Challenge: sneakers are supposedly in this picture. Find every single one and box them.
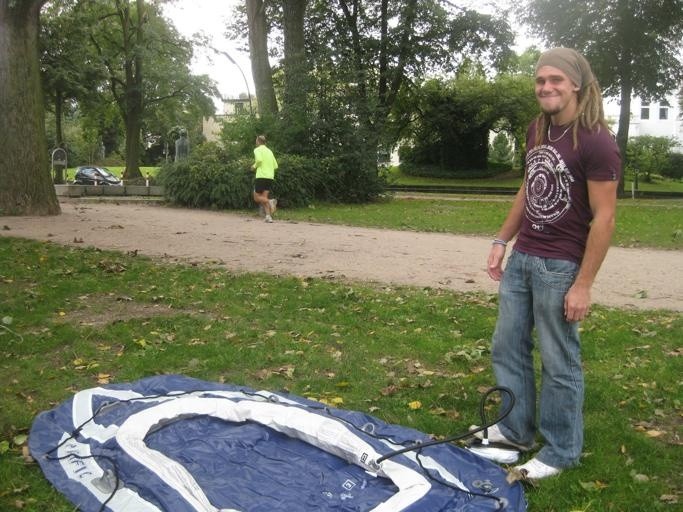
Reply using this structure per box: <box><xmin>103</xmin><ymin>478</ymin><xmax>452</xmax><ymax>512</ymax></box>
<box><xmin>514</xmin><ymin>458</ymin><xmax>561</xmax><ymax>481</ymax></box>
<box><xmin>469</xmin><ymin>423</ymin><xmax>530</xmax><ymax>452</ymax></box>
<box><xmin>265</xmin><ymin>216</ymin><xmax>273</xmax><ymax>223</ymax></box>
<box><xmin>269</xmin><ymin>199</ymin><xmax>277</xmax><ymax>214</ymax></box>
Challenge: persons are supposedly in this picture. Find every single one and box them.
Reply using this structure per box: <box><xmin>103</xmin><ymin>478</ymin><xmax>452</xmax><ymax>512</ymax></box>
<box><xmin>251</xmin><ymin>136</ymin><xmax>278</xmax><ymax>223</ymax></box>
<box><xmin>468</xmin><ymin>47</ymin><xmax>622</xmax><ymax>479</ymax></box>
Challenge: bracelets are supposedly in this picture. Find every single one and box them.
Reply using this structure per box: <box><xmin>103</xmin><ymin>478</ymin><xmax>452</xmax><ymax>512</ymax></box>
<box><xmin>492</xmin><ymin>238</ymin><xmax>508</xmax><ymax>246</ymax></box>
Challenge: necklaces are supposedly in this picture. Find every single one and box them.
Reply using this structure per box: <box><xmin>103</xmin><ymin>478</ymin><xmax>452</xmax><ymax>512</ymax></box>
<box><xmin>547</xmin><ymin>119</ymin><xmax>576</xmax><ymax>143</ymax></box>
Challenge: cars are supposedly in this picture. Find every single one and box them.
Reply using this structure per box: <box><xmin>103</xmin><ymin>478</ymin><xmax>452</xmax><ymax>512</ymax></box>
<box><xmin>73</xmin><ymin>166</ymin><xmax>123</xmax><ymax>187</ymax></box>
